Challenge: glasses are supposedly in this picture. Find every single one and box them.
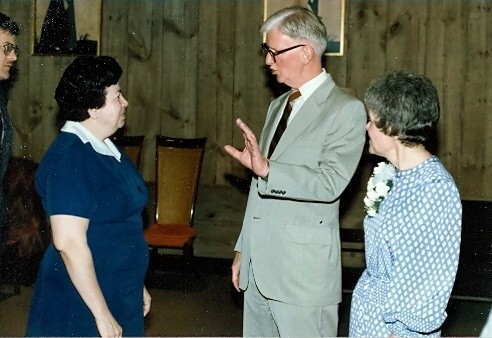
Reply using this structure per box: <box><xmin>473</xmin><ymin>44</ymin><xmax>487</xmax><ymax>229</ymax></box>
<box><xmin>0</xmin><ymin>43</ymin><xmax>20</xmax><ymax>57</ymax></box>
<box><xmin>261</xmin><ymin>41</ymin><xmax>308</xmax><ymax>63</ymax></box>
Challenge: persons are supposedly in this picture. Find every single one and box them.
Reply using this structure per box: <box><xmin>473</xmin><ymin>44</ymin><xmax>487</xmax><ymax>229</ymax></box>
<box><xmin>350</xmin><ymin>69</ymin><xmax>462</xmax><ymax>337</ymax></box>
<box><xmin>224</xmin><ymin>5</ymin><xmax>366</xmax><ymax>337</ymax></box>
<box><xmin>25</xmin><ymin>56</ymin><xmax>152</xmax><ymax>338</ymax></box>
<box><xmin>0</xmin><ymin>11</ymin><xmax>21</xmax><ymax>183</ymax></box>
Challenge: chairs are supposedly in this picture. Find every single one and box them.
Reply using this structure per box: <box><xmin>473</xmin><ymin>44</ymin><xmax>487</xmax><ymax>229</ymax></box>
<box><xmin>143</xmin><ymin>135</ymin><xmax>207</xmax><ymax>283</ymax></box>
<box><xmin>118</xmin><ymin>135</ymin><xmax>146</xmax><ymax>167</ymax></box>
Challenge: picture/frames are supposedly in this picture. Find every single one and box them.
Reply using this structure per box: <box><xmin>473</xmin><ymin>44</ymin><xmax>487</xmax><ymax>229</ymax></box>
<box><xmin>263</xmin><ymin>0</ymin><xmax>344</xmax><ymax>56</ymax></box>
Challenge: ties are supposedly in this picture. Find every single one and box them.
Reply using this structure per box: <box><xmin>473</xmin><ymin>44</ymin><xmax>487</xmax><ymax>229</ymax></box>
<box><xmin>267</xmin><ymin>89</ymin><xmax>301</xmax><ymax>158</ymax></box>
